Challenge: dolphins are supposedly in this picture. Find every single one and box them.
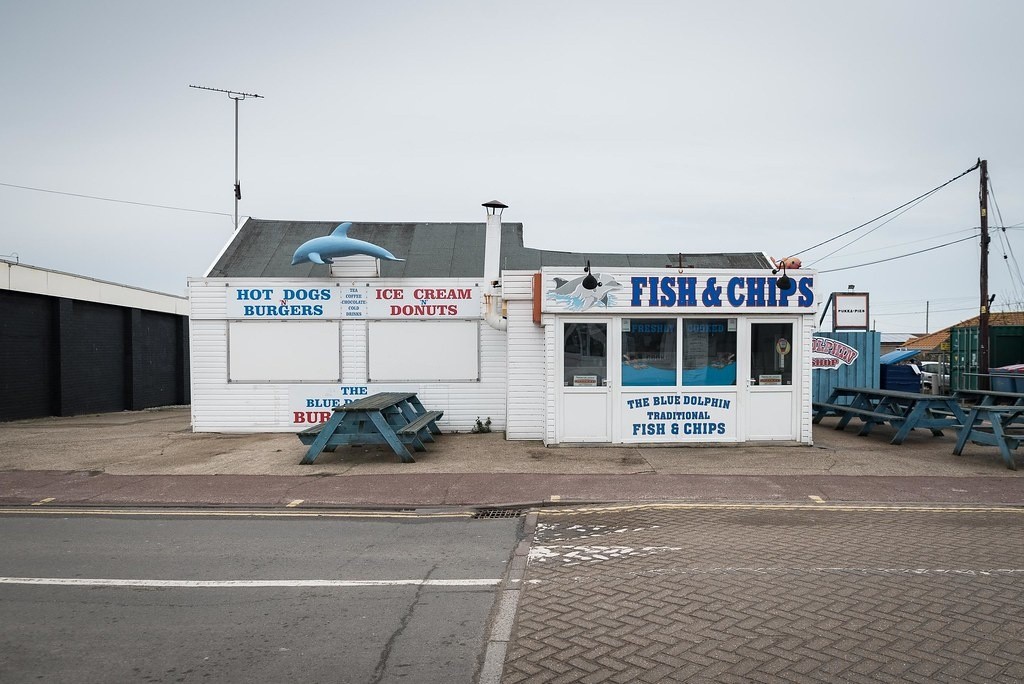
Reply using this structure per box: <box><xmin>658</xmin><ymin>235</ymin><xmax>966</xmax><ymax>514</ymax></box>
<box><xmin>290</xmin><ymin>222</ymin><xmax>406</xmax><ymax>266</ymax></box>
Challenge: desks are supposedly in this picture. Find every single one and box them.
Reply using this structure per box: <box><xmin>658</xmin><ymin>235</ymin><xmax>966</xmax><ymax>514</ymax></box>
<box><xmin>952</xmin><ymin>406</ymin><xmax>1024</xmax><ymax>471</ymax></box>
<box><xmin>811</xmin><ymin>386</ymin><xmax>967</xmax><ymax>445</ymax></box>
<box><xmin>953</xmin><ymin>389</ymin><xmax>1024</xmax><ymax>425</ymax></box>
<box><xmin>298</xmin><ymin>392</ymin><xmax>443</xmax><ymax>465</ymax></box>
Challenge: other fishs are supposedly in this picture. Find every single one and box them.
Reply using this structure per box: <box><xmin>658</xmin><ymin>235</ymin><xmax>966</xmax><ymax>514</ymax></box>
<box><xmin>770</xmin><ymin>257</ymin><xmax>801</xmax><ymax>270</ymax></box>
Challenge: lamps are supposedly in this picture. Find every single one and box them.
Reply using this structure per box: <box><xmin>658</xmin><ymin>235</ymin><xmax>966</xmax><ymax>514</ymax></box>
<box><xmin>772</xmin><ymin>260</ymin><xmax>791</xmax><ymax>290</ymax></box>
<box><xmin>582</xmin><ymin>259</ymin><xmax>598</xmax><ymax>290</ymax></box>
<box><xmin>848</xmin><ymin>285</ymin><xmax>855</xmax><ymax>292</ymax></box>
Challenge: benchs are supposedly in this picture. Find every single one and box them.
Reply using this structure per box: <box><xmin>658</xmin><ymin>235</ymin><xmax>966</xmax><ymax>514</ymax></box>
<box><xmin>396</xmin><ymin>410</ymin><xmax>444</xmax><ymax>443</ymax></box>
<box><xmin>296</xmin><ymin>421</ymin><xmax>326</xmax><ymax>446</ymax></box>
<box><xmin>812</xmin><ymin>402</ymin><xmax>905</xmax><ymax>421</ymax></box>
<box><xmin>870</xmin><ymin>402</ymin><xmax>1024</xmax><ymax>440</ymax></box>
